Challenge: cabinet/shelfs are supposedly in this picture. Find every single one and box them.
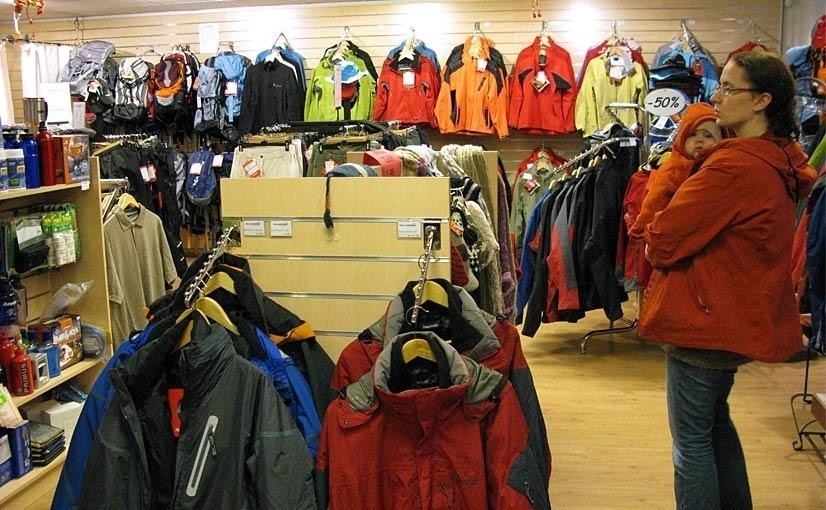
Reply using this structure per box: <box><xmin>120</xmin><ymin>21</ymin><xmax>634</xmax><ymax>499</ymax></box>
<box><xmin>0</xmin><ymin>157</ymin><xmax>108</xmax><ymax>510</ymax></box>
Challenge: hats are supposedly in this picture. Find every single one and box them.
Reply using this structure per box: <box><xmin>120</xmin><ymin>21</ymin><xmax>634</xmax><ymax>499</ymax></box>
<box><xmin>697</xmin><ymin>120</ymin><xmax>722</xmax><ymax>140</ymax></box>
<box><xmin>325</xmin><ymin>60</ymin><xmax>370</xmax><ymax>85</ymax></box>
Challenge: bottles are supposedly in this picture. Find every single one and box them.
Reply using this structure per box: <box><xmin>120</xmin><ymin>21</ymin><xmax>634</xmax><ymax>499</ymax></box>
<box><xmin>1</xmin><ymin>272</ymin><xmax>60</xmax><ymax>397</ymax></box>
<box><xmin>0</xmin><ymin>121</ymin><xmax>65</xmax><ymax>191</ymax></box>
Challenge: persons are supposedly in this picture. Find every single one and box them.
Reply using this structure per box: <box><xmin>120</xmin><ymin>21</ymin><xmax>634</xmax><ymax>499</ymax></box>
<box><xmin>626</xmin><ymin>102</ymin><xmax>731</xmax><ymax>241</ymax></box>
<box><xmin>642</xmin><ymin>49</ymin><xmax>801</xmax><ymax>510</ymax></box>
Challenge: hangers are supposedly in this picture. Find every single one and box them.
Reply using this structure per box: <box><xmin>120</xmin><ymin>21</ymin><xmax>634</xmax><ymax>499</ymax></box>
<box><xmin>159</xmin><ymin>258</ymin><xmax>247</xmax><ymax>357</ymax></box>
<box><xmin>101</xmin><ymin>179</ymin><xmax>147</xmax><ymax>223</ymax></box>
<box><xmin>395</xmin><ymin>247</ymin><xmax>456</xmax><ymax>374</ymax></box>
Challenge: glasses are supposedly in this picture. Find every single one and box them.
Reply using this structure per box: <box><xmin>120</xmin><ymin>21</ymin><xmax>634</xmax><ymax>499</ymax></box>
<box><xmin>716</xmin><ymin>84</ymin><xmax>760</xmax><ymax>95</ymax></box>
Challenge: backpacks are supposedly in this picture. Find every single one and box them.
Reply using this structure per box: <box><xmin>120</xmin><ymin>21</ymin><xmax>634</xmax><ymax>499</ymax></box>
<box><xmin>173</xmin><ymin>146</ymin><xmax>225</xmax><ymax>235</ymax></box>
<box><xmin>114</xmin><ymin>40</ymin><xmax>228</xmax><ymax>133</ymax></box>
